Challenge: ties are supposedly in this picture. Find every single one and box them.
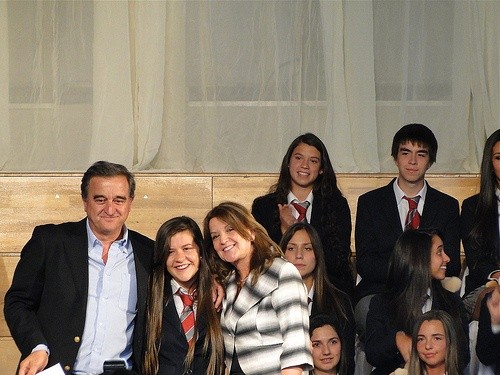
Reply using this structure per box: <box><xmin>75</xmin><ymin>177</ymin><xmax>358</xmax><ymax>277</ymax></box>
<box><xmin>402</xmin><ymin>195</ymin><xmax>421</xmax><ymax>231</ymax></box>
<box><xmin>175</xmin><ymin>288</ymin><xmax>200</xmax><ymax>352</ymax></box>
<box><xmin>290</xmin><ymin>199</ymin><xmax>310</xmax><ymax>224</ymax></box>
<box><xmin>308</xmin><ymin>297</ymin><xmax>311</xmax><ymax>305</ymax></box>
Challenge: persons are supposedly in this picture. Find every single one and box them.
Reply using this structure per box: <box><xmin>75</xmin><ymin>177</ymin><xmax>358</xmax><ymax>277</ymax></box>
<box><xmin>365</xmin><ymin>226</ymin><xmax>469</xmax><ymax>374</ymax></box>
<box><xmin>251</xmin><ymin>133</ymin><xmax>352</xmax><ymax>290</ymax></box>
<box><xmin>3</xmin><ymin>160</ymin><xmax>224</xmax><ymax>375</ymax></box>
<box><xmin>306</xmin><ymin>314</ymin><xmax>347</xmax><ymax>375</ymax></box>
<box><xmin>459</xmin><ymin>130</ymin><xmax>500</xmax><ymax>296</ymax></box>
<box><xmin>137</xmin><ymin>214</ymin><xmax>222</xmax><ymax>375</ymax></box>
<box><xmin>202</xmin><ymin>200</ymin><xmax>314</xmax><ymax>375</ymax></box>
<box><xmin>349</xmin><ymin>123</ymin><xmax>462</xmax><ymax>300</ymax></box>
<box><xmin>277</xmin><ymin>223</ymin><xmax>358</xmax><ymax>375</ymax></box>
<box><xmin>392</xmin><ymin>311</ymin><xmax>460</xmax><ymax>375</ymax></box>
